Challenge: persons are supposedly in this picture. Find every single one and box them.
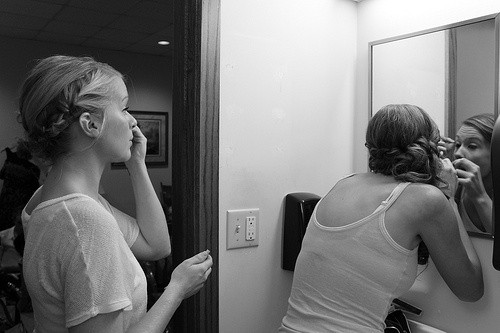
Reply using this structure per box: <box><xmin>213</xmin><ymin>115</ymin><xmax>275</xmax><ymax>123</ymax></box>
<box><xmin>437</xmin><ymin>113</ymin><xmax>496</xmax><ymax>235</ymax></box>
<box><xmin>17</xmin><ymin>55</ymin><xmax>212</xmax><ymax>333</ymax></box>
<box><xmin>277</xmin><ymin>103</ymin><xmax>483</xmax><ymax>333</ymax></box>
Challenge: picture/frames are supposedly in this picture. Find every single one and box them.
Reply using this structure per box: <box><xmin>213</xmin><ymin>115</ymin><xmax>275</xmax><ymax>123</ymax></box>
<box><xmin>111</xmin><ymin>110</ymin><xmax>168</xmax><ymax>168</ymax></box>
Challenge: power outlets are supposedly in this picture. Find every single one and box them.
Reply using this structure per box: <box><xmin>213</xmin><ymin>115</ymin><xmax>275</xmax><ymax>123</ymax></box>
<box><xmin>226</xmin><ymin>208</ymin><xmax>260</xmax><ymax>250</ymax></box>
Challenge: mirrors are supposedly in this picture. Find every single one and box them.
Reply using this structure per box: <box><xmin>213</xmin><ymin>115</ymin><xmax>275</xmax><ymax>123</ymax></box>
<box><xmin>367</xmin><ymin>11</ymin><xmax>500</xmax><ymax>244</ymax></box>
